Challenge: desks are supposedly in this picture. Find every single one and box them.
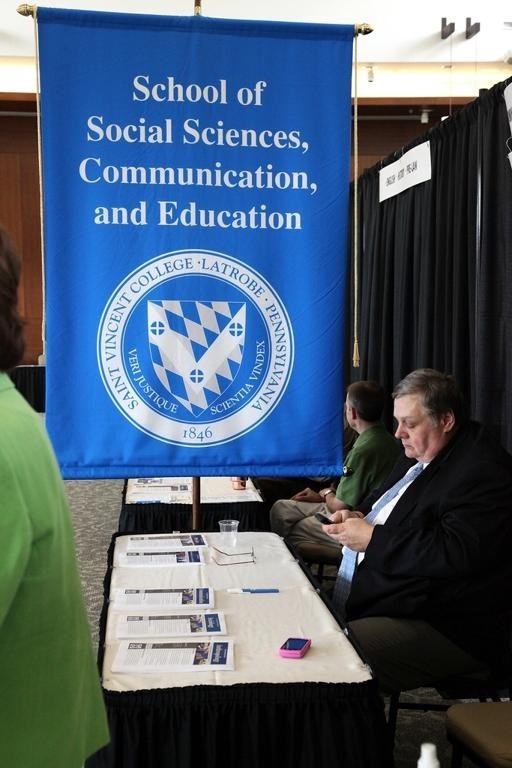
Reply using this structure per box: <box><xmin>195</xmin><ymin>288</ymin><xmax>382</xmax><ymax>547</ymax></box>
<box><xmin>97</xmin><ymin>530</ymin><xmax>390</xmax><ymax>768</ymax></box>
<box><xmin>118</xmin><ymin>475</ymin><xmax>265</xmax><ymax>527</ymax></box>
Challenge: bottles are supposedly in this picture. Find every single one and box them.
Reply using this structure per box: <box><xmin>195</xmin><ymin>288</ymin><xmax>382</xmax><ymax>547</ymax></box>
<box><xmin>416</xmin><ymin>740</ymin><xmax>441</xmax><ymax>767</ymax></box>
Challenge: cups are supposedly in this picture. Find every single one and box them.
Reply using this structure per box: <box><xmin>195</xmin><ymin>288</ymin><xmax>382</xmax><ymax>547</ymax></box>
<box><xmin>218</xmin><ymin>518</ymin><xmax>239</xmax><ymax>544</ymax></box>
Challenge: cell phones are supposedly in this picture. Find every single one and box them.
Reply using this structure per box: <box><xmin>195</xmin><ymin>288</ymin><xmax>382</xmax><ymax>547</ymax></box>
<box><xmin>279</xmin><ymin>637</ymin><xmax>311</xmax><ymax>659</ymax></box>
<box><xmin>315</xmin><ymin>513</ymin><xmax>335</xmax><ymax>525</ymax></box>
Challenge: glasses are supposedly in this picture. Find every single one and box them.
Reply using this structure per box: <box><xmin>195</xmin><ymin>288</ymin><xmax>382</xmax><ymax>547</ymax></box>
<box><xmin>211</xmin><ymin>546</ymin><xmax>256</xmax><ymax>565</ymax></box>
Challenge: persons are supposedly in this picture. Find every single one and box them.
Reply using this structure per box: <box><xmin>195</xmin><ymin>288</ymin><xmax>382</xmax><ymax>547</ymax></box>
<box><xmin>0</xmin><ymin>228</ymin><xmax>112</xmax><ymax>768</ymax></box>
<box><xmin>322</xmin><ymin>367</ymin><xmax>511</xmax><ymax>709</ymax></box>
<box><xmin>267</xmin><ymin>379</ymin><xmax>406</xmax><ymax>546</ymax></box>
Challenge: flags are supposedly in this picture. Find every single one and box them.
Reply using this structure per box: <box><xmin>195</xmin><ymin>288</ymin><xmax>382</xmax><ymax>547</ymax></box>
<box><xmin>36</xmin><ymin>7</ymin><xmax>357</xmax><ymax>480</ymax></box>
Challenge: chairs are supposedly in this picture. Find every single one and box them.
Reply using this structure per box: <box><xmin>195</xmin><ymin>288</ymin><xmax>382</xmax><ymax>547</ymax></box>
<box><xmin>300</xmin><ymin>541</ymin><xmax>342</xmax><ymax>589</ymax></box>
<box><xmin>391</xmin><ymin>684</ymin><xmax>511</xmax><ymax>766</ymax></box>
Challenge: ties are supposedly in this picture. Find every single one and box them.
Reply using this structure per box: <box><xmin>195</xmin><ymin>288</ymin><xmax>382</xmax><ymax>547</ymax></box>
<box><xmin>333</xmin><ymin>464</ymin><xmax>424</xmax><ymax>614</ymax></box>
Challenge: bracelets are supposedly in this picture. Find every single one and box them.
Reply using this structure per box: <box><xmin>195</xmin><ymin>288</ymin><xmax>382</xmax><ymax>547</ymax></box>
<box><xmin>322</xmin><ymin>486</ymin><xmax>334</xmax><ymax>497</ymax></box>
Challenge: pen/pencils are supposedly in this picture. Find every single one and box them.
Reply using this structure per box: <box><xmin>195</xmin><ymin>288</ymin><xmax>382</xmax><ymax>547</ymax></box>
<box><xmin>227</xmin><ymin>589</ymin><xmax>278</xmax><ymax>594</ymax></box>
